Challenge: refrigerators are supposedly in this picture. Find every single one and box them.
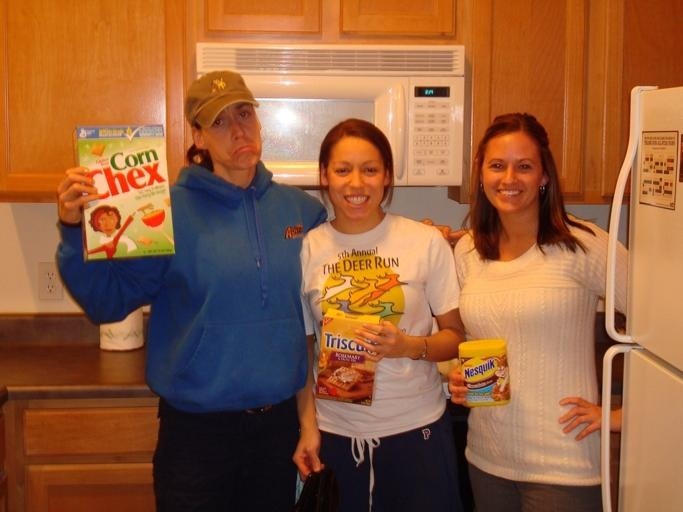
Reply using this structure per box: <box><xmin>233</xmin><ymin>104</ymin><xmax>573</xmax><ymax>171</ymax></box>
<box><xmin>602</xmin><ymin>84</ymin><xmax>683</xmax><ymax>512</ymax></box>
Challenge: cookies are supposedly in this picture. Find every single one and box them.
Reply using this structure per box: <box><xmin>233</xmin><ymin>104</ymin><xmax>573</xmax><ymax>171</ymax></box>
<box><xmin>327</xmin><ymin>365</ymin><xmax>362</xmax><ymax>391</ymax></box>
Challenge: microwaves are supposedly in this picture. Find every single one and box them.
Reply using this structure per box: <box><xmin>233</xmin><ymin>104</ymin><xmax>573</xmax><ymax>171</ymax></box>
<box><xmin>194</xmin><ymin>43</ymin><xmax>463</xmax><ymax>187</ymax></box>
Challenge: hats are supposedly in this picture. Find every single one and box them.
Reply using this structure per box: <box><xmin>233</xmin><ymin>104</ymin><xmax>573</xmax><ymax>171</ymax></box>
<box><xmin>183</xmin><ymin>70</ymin><xmax>261</xmax><ymax>129</ymax></box>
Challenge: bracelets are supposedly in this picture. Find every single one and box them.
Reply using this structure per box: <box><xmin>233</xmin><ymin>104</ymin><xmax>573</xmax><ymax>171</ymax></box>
<box><xmin>413</xmin><ymin>335</ymin><xmax>430</xmax><ymax>362</ymax></box>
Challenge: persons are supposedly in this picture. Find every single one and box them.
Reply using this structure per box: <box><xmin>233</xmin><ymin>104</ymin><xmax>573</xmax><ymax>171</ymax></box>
<box><xmin>54</xmin><ymin>70</ymin><xmax>468</xmax><ymax>511</ymax></box>
<box><xmin>452</xmin><ymin>110</ymin><xmax>628</xmax><ymax>512</ymax></box>
<box><xmin>291</xmin><ymin>118</ymin><xmax>466</xmax><ymax>512</ymax></box>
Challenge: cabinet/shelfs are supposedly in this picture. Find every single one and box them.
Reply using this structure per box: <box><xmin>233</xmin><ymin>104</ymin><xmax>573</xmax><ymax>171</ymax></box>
<box><xmin>185</xmin><ymin>2</ymin><xmax>466</xmax><ymax>40</ymax></box>
<box><xmin>602</xmin><ymin>1</ymin><xmax>682</xmax><ymax>206</ymax></box>
<box><xmin>1</xmin><ymin>0</ymin><xmax>185</xmax><ymax>198</ymax></box>
<box><xmin>467</xmin><ymin>1</ymin><xmax>605</xmax><ymax>206</ymax></box>
<box><xmin>3</xmin><ymin>394</ymin><xmax>163</xmax><ymax>512</ymax></box>
